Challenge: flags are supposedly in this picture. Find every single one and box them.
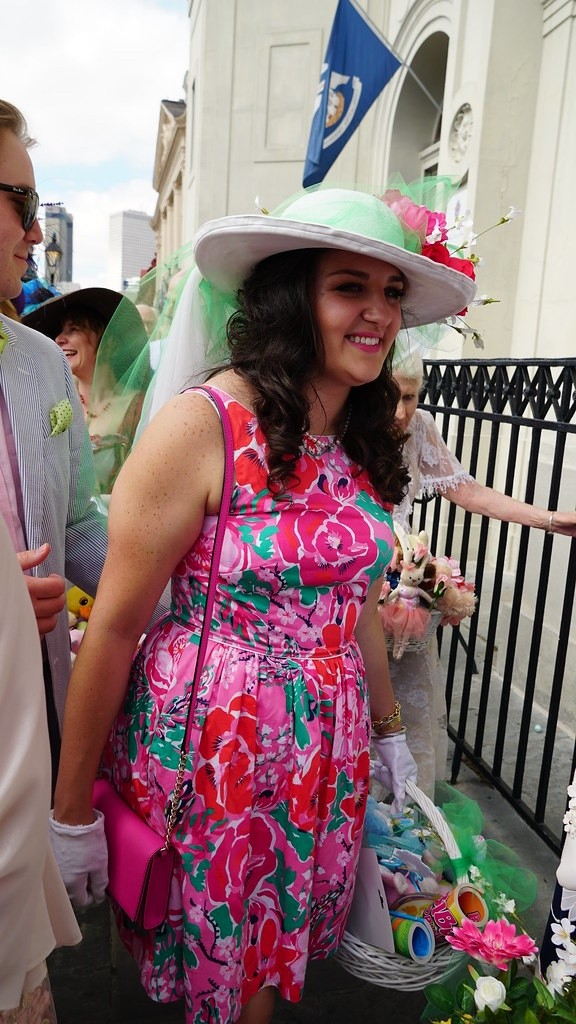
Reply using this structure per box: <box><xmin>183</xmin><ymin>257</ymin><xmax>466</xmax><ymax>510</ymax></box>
<box><xmin>303</xmin><ymin>0</ymin><xmax>401</xmax><ymax>189</ymax></box>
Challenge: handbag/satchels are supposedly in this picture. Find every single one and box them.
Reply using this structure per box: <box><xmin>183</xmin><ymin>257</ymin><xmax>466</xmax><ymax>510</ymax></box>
<box><xmin>92</xmin><ymin>779</ymin><xmax>172</xmax><ymax>933</ymax></box>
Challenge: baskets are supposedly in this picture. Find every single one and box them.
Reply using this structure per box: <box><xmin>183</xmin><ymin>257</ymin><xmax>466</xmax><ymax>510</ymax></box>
<box><xmin>379</xmin><ymin>521</ymin><xmax>445</xmax><ymax>651</ymax></box>
<box><xmin>336</xmin><ymin>762</ymin><xmax>473</xmax><ymax>990</ymax></box>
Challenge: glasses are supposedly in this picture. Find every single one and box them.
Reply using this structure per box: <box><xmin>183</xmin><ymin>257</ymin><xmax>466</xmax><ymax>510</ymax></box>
<box><xmin>0</xmin><ymin>183</ymin><xmax>39</xmax><ymax>232</ymax></box>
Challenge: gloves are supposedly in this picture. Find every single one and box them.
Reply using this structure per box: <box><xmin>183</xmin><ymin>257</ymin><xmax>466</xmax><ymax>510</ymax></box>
<box><xmin>50</xmin><ymin>810</ymin><xmax>109</xmax><ymax>909</ymax></box>
<box><xmin>374</xmin><ymin>734</ymin><xmax>419</xmax><ymax>813</ymax></box>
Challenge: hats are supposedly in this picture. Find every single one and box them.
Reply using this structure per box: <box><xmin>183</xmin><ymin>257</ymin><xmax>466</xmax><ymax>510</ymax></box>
<box><xmin>19</xmin><ymin>287</ymin><xmax>149</xmax><ymax>389</ymax></box>
<box><xmin>194</xmin><ymin>189</ymin><xmax>478</xmax><ymax>330</ymax></box>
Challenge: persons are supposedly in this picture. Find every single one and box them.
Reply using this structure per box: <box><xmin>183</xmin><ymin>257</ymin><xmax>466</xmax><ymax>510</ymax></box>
<box><xmin>0</xmin><ymin>97</ymin><xmax>576</xmax><ymax>1024</ymax></box>
